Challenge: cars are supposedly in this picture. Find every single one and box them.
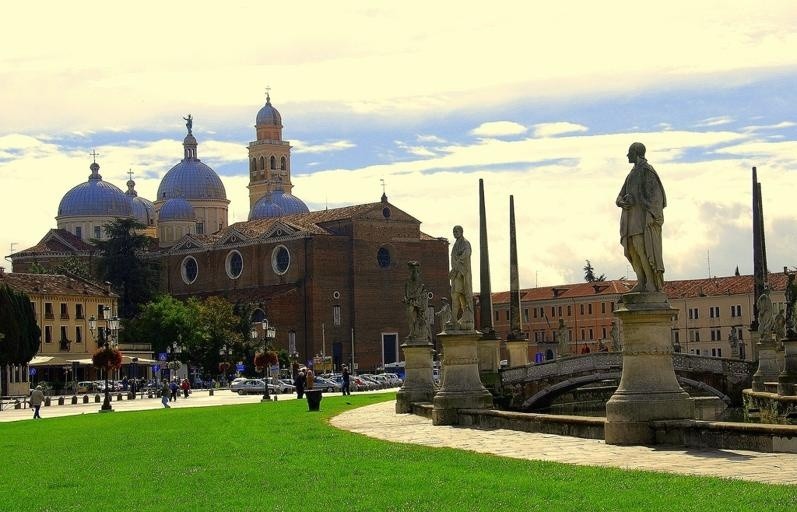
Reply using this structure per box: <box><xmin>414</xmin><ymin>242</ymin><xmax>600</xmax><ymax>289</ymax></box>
<box><xmin>227</xmin><ymin>376</ymin><xmax>296</xmax><ymax>394</ymax></box>
<box><xmin>78</xmin><ymin>377</ymin><xmax>219</xmax><ymax>393</ymax></box>
<box><xmin>304</xmin><ymin>371</ymin><xmax>402</xmax><ymax>393</ymax></box>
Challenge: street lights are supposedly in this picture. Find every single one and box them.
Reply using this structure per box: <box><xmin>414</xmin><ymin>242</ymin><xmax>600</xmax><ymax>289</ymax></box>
<box><xmin>62</xmin><ymin>365</ymin><xmax>72</xmax><ymax>395</ymax></box>
<box><xmin>288</xmin><ymin>344</ymin><xmax>300</xmax><ymax>366</ymax></box>
<box><xmin>250</xmin><ymin>318</ymin><xmax>276</xmax><ymax>401</ymax></box>
<box><xmin>219</xmin><ymin>343</ymin><xmax>233</xmax><ymax>387</ymax></box>
<box><xmin>87</xmin><ymin>302</ymin><xmax>117</xmax><ymax>411</ymax></box>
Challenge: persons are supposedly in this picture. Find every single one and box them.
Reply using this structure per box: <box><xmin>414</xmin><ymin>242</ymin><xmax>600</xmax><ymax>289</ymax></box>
<box><xmin>551</xmin><ymin>318</ymin><xmax>570</xmax><ymax>355</ymax></box>
<box><xmin>295</xmin><ymin>363</ymin><xmax>350</xmax><ymax>399</ymax></box>
<box><xmin>445</xmin><ymin>225</ymin><xmax>474</xmax><ymax>324</ymax></box>
<box><xmin>30</xmin><ymin>385</ymin><xmax>46</xmax><ymax>419</ymax></box>
<box><xmin>756</xmin><ymin>272</ymin><xmax>797</xmax><ymax>342</ymax></box>
<box><xmin>402</xmin><ymin>260</ymin><xmax>430</xmax><ymax>340</ymax></box>
<box><xmin>545</xmin><ymin>338</ymin><xmax>608</xmax><ymax>360</ymax></box>
<box><xmin>615</xmin><ymin>143</ymin><xmax>668</xmax><ymax>292</ymax></box>
<box><xmin>609</xmin><ymin>321</ymin><xmax>619</xmax><ymax>350</ymax></box>
<box><xmin>434</xmin><ymin>297</ymin><xmax>451</xmax><ymax>332</ymax></box>
<box><xmin>122</xmin><ymin>376</ymin><xmax>191</xmax><ymax>408</ymax></box>
